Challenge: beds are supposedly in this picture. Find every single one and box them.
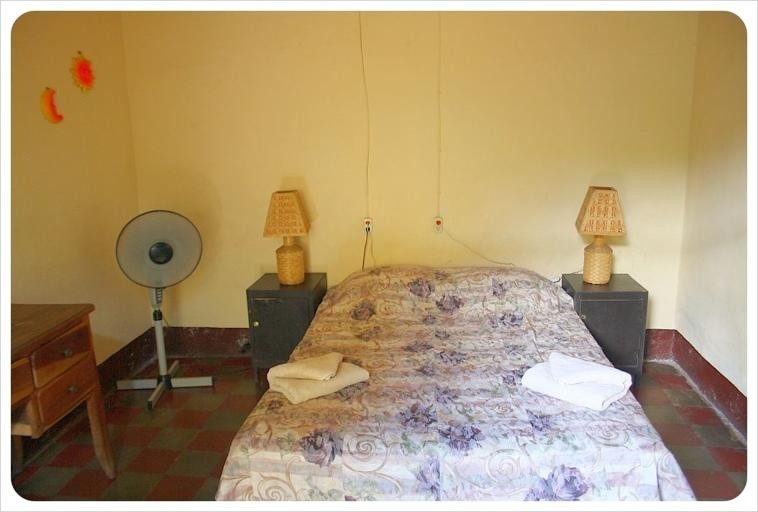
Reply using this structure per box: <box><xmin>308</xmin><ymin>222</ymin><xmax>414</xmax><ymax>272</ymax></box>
<box><xmin>215</xmin><ymin>267</ymin><xmax>698</xmax><ymax>500</ymax></box>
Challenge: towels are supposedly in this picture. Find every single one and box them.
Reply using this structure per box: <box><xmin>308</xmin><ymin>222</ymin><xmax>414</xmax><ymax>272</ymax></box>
<box><xmin>265</xmin><ymin>350</ymin><xmax>373</xmax><ymax>403</ymax></box>
<box><xmin>515</xmin><ymin>353</ymin><xmax>635</xmax><ymax>410</ymax></box>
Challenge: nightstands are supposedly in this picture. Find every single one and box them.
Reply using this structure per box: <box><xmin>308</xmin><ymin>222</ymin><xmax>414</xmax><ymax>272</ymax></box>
<box><xmin>563</xmin><ymin>274</ymin><xmax>648</xmax><ymax>385</ymax></box>
<box><xmin>247</xmin><ymin>271</ymin><xmax>327</xmax><ymax>382</ymax></box>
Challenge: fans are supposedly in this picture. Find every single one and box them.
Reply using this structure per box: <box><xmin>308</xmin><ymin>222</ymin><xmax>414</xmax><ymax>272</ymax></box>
<box><xmin>114</xmin><ymin>207</ymin><xmax>214</xmax><ymax>410</ymax></box>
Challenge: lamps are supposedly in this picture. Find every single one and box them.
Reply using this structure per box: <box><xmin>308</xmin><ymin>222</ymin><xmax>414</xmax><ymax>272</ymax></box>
<box><xmin>576</xmin><ymin>186</ymin><xmax>625</xmax><ymax>284</ymax></box>
<box><xmin>263</xmin><ymin>188</ymin><xmax>312</xmax><ymax>288</ymax></box>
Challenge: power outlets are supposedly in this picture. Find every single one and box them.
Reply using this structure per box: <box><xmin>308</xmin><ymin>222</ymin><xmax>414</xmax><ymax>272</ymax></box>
<box><xmin>434</xmin><ymin>217</ymin><xmax>443</xmax><ymax>234</ymax></box>
<box><xmin>363</xmin><ymin>218</ymin><xmax>373</xmax><ymax>234</ymax></box>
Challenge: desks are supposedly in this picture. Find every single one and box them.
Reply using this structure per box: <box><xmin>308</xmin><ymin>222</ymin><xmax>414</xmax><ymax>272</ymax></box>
<box><xmin>12</xmin><ymin>303</ymin><xmax>118</xmax><ymax>490</ymax></box>
